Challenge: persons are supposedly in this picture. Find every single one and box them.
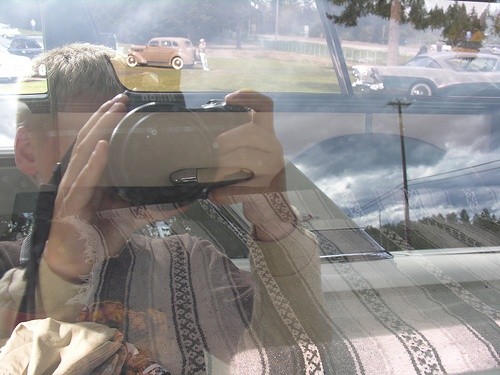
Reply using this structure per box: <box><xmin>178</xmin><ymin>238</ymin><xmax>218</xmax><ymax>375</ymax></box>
<box><xmin>198</xmin><ymin>39</ymin><xmax>209</xmax><ymax>71</ymax></box>
<box><xmin>1</xmin><ymin>43</ymin><xmax>328</xmax><ymax>375</ymax></box>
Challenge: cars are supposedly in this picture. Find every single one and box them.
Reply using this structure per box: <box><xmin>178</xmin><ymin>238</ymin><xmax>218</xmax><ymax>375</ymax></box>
<box><xmin>127</xmin><ymin>36</ymin><xmax>197</xmax><ymax>70</ymax></box>
<box><xmin>8</xmin><ymin>37</ymin><xmax>44</xmax><ymax>58</ymax></box>
<box><xmin>353</xmin><ymin>52</ymin><xmax>500</xmax><ymax>95</ymax></box>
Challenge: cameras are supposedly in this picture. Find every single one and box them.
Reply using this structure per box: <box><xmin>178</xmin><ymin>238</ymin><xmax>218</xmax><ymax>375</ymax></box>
<box><xmin>103</xmin><ymin>94</ymin><xmax>252</xmax><ymax>206</ymax></box>
